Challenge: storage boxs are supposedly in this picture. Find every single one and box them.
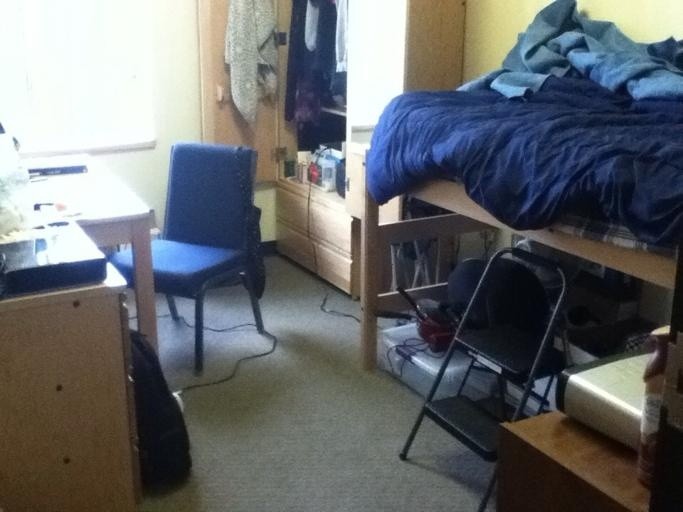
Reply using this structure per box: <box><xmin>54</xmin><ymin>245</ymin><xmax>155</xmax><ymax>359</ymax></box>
<box><xmin>375</xmin><ymin>322</ymin><xmax>470</xmax><ymax>401</ymax></box>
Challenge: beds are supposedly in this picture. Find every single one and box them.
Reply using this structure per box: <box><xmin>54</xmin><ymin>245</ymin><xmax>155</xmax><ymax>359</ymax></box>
<box><xmin>361</xmin><ymin>0</ymin><xmax>683</xmax><ymax>421</ymax></box>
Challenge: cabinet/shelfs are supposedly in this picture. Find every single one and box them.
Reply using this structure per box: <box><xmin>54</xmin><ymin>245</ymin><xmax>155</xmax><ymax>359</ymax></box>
<box><xmin>0</xmin><ymin>261</ymin><xmax>142</xmax><ymax>511</ymax></box>
<box><xmin>200</xmin><ymin>0</ymin><xmax>464</xmax><ymax>301</ymax></box>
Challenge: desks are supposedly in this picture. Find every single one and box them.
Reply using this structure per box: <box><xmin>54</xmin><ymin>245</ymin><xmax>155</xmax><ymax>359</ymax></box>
<box><xmin>500</xmin><ymin>409</ymin><xmax>678</xmax><ymax>511</ymax></box>
<box><xmin>0</xmin><ymin>153</ymin><xmax>159</xmax><ymax>357</ymax></box>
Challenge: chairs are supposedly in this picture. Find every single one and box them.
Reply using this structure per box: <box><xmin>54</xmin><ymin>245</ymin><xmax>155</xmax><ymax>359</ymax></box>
<box><xmin>111</xmin><ymin>143</ymin><xmax>264</xmax><ymax>374</ymax></box>
